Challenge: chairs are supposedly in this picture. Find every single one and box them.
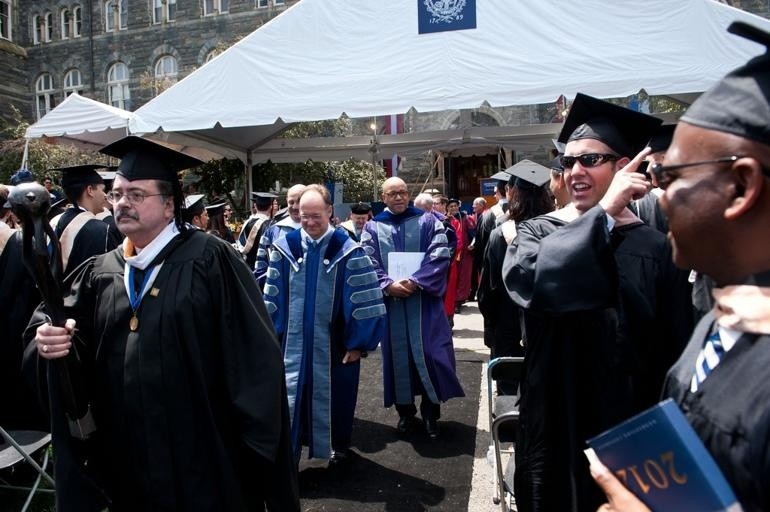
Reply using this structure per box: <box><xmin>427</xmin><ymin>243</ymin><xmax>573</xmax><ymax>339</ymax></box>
<box><xmin>0</xmin><ymin>426</ymin><xmax>56</xmax><ymax>512</ymax></box>
<box><xmin>487</xmin><ymin>356</ymin><xmax>526</xmax><ymax>512</ymax></box>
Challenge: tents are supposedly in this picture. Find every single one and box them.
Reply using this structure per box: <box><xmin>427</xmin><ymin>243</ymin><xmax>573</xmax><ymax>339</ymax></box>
<box><xmin>19</xmin><ymin>93</ymin><xmax>131</xmax><ymax>170</ymax></box>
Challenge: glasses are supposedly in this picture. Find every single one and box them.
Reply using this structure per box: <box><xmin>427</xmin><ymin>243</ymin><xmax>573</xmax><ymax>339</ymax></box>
<box><xmin>652</xmin><ymin>155</ymin><xmax>737</xmax><ymax>191</ymax></box>
<box><xmin>105</xmin><ymin>190</ymin><xmax>165</xmax><ymax>207</ymax></box>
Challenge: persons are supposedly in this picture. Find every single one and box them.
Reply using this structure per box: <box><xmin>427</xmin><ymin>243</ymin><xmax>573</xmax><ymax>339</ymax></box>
<box><xmin>186</xmin><ymin>158</ymin><xmax>552</xmax><ymax>481</ymax></box>
<box><xmin>0</xmin><ymin>136</ymin><xmax>301</xmax><ymax>512</ymax></box>
<box><xmin>502</xmin><ymin>21</ymin><xmax>769</xmax><ymax>512</ymax></box>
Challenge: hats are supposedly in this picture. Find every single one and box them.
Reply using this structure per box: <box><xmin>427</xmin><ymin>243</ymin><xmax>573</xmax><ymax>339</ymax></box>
<box><xmin>249</xmin><ymin>192</ymin><xmax>279</xmax><ymax>219</ymax></box>
<box><xmin>490</xmin><ymin>171</ymin><xmax>511</xmax><ymax>181</ymax></box>
<box><xmin>677</xmin><ymin>20</ymin><xmax>769</xmax><ymax>142</ymax></box>
<box><xmin>180</xmin><ymin>194</ymin><xmax>227</xmax><ymax>216</ymax></box>
<box><xmin>504</xmin><ymin>159</ymin><xmax>550</xmax><ymax>188</ymax></box>
<box><xmin>446</xmin><ymin>198</ymin><xmax>460</xmax><ymax>207</ymax></box>
<box><xmin>550</xmin><ymin>92</ymin><xmax>664</xmax><ymax>171</ymax></box>
<box><xmin>350</xmin><ymin>203</ymin><xmax>371</xmax><ymax>215</ymax></box>
<box><xmin>44</xmin><ymin>165</ymin><xmax>108</xmax><ymax>185</ymax></box>
<box><xmin>98</xmin><ymin>135</ymin><xmax>205</xmax><ymax>234</ymax></box>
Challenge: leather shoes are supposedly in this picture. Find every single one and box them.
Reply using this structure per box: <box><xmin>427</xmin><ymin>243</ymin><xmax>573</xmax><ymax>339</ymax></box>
<box><xmin>455</xmin><ymin>307</ymin><xmax>460</xmax><ymax>313</ymax></box>
<box><xmin>330</xmin><ymin>450</ymin><xmax>346</xmax><ymax>468</ymax></box>
<box><xmin>423</xmin><ymin>418</ymin><xmax>438</xmax><ymax>439</ymax></box>
<box><xmin>398</xmin><ymin>410</ymin><xmax>418</xmax><ymax>434</ymax></box>
